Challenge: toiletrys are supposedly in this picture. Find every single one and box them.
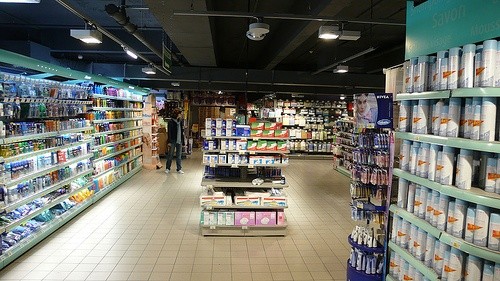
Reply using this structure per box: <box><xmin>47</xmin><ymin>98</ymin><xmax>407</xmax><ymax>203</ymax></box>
<box><xmin>389</xmin><ymin>249</ymin><xmax>430</xmax><ymax>281</ymax></box>
<box><xmin>396</xmin><ymin>177</ymin><xmax>500</xmax><ymax>251</ymax></box>
<box><xmin>391</xmin><ymin>213</ymin><xmax>500</xmax><ymax>281</ymax></box>
<box><xmin>399</xmin><ymin>138</ymin><xmax>500</xmax><ymax>194</ymax></box>
<box><xmin>402</xmin><ymin>40</ymin><xmax>500</xmax><ymax>93</ymax></box>
<box><xmin>397</xmin><ymin>97</ymin><xmax>497</xmax><ymax>142</ymax></box>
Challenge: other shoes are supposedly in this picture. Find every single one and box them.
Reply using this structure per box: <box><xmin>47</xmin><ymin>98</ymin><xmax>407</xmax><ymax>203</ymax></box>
<box><xmin>177</xmin><ymin>170</ymin><xmax>184</xmax><ymax>174</ymax></box>
<box><xmin>165</xmin><ymin>169</ymin><xmax>170</xmax><ymax>174</ymax></box>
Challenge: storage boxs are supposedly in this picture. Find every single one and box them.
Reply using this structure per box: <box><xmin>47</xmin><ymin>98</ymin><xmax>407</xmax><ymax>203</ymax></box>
<box><xmin>200</xmin><ymin>119</ymin><xmax>290</xmax><ymax>226</ymax></box>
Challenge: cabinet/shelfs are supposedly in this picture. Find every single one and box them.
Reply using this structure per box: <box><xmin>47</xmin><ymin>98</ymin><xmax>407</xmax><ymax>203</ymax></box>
<box><xmin>0</xmin><ymin>49</ymin><xmax>193</xmax><ymax>270</ymax></box>
<box><xmin>199</xmin><ymin>107</ymin><xmax>236</xmax><ymax>126</ymax></box>
<box><xmin>248</xmin><ymin>67</ymin><xmax>500</xmax><ymax>281</ymax></box>
<box><xmin>200</xmin><ymin>129</ymin><xmax>290</xmax><ymax>236</ymax></box>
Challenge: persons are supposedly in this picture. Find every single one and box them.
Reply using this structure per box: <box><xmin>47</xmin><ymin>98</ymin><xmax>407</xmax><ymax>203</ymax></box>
<box><xmin>355</xmin><ymin>94</ymin><xmax>375</xmax><ymax>124</ymax></box>
<box><xmin>164</xmin><ymin>106</ymin><xmax>185</xmax><ymax>174</ymax></box>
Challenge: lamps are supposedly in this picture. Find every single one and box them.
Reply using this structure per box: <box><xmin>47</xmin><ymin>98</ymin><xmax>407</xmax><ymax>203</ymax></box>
<box><xmin>333</xmin><ymin>69</ymin><xmax>337</xmax><ymax>72</ymax></box>
<box><xmin>124</xmin><ymin>48</ymin><xmax>138</xmax><ymax>59</ymax></box>
<box><xmin>336</xmin><ymin>65</ymin><xmax>349</xmax><ymax>72</ymax></box>
<box><xmin>318</xmin><ymin>21</ymin><xmax>360</xmax><ymax>40</ymax></box>
<box><xmin>142</xmin><ymin>63</ymin><xmax>156</xmax><ymax>75</ymax></box>
<box><xmin>171</xmin><ymin>82</ymin><xmax>180</xmax><ymax>86</ymax></box>
<box><xmin>70</xmin><ymin>21</ymin><xmax>102</xmax><ymax>45</ymax></box>
